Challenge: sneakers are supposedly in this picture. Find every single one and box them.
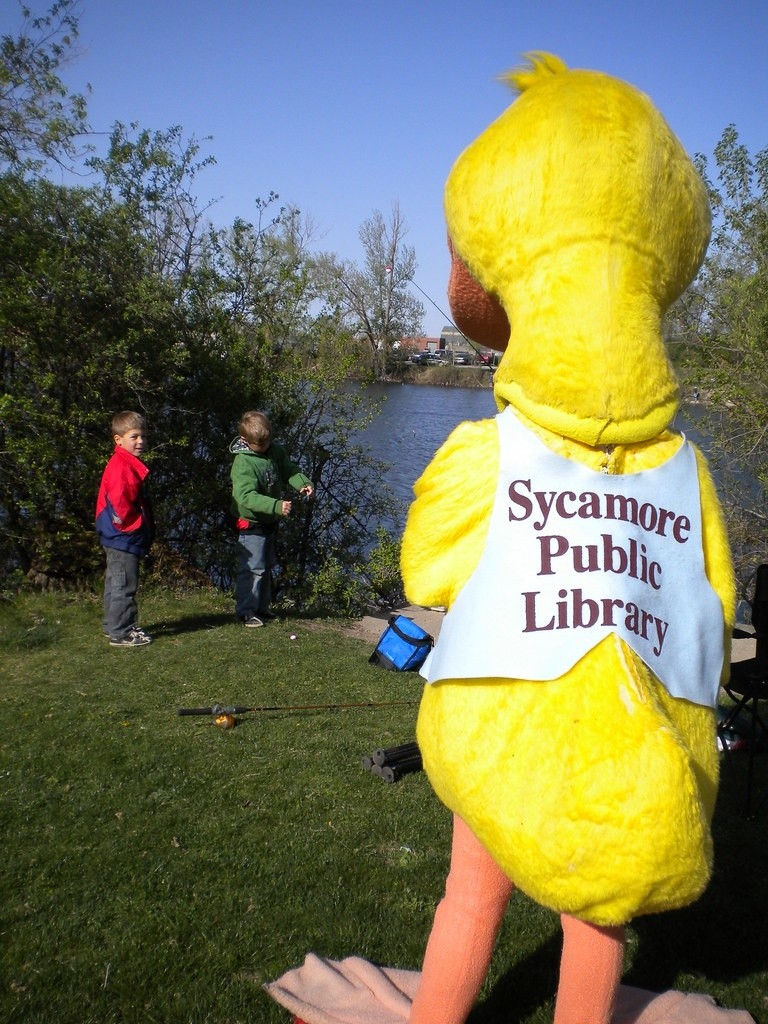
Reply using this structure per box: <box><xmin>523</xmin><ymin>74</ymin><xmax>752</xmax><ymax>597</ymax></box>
<box><xmin>102</xmin><ymin>628</ymin><xmax>146</xmax><ymax>638</ymax></box>
<box><xmin>255</xmin><ymin>607</ymin><xmax>280</xmax><ymax>621</ymax></box>
<box><xmin>108</xmin><ymin>631</ymin><xmax>152</xmax><ymax>647</ymax></box>
<box><xmin>239</xmin><ymin>613</ymin><xmax>264</xmax><ymax>628</ymax></box>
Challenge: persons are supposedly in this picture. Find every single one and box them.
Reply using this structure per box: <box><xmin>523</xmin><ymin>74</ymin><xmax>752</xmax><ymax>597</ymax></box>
<box><xmin>94</xmin><ymin>410</ymin><xmax>152</xmax><ymax>647</ymax></box>
<box><xmin>229</xmin><ymin>411</ymin><xmax>315</xmax><ymax>627</ymax></box>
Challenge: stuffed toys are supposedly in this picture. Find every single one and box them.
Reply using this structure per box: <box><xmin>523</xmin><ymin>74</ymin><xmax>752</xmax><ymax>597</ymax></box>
<box><xmin>260</xmin><ymin>49</ymin><xmax>756</xmax><ymax>1024</ymax></box>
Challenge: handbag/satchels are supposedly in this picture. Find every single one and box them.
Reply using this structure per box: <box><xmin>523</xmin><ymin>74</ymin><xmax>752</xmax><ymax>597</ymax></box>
<box><xmin>368</xmin><ymin>614</ymin><xmax>435</xmax><ymax>671</ymax></box>
